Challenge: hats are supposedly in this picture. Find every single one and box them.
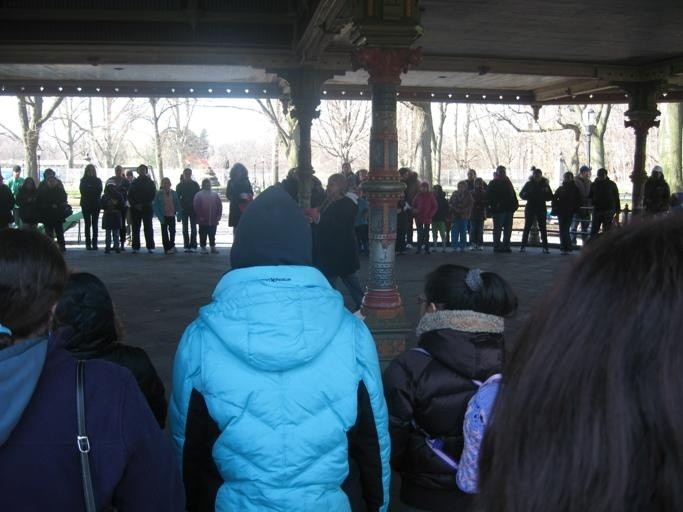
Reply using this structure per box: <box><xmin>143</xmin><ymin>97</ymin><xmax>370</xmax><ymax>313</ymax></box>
<box><xmin>231</xmin><ymin>184</ymin><xmax>313</xmax><ymax>266</ymax></box>
<box><xmin>580</xmin><ymin>166</ymin><xmax>591</xmax><ymax>173</ymax></box>
<box><xmin>653</xmin><ymin>166</ymin><xmax>662</xmax><ymax>173</ymax></box>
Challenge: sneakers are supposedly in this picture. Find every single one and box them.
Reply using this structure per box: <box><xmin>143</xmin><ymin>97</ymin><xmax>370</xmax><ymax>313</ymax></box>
<box><xmin>470</xmin><ymin>243</ymin><xmax>483</xmax><ymax>250</ymax></box>
<box><xmin>184</xmin><ymin>248</ymin><xmax>195</xmax><ymax>252</ymax></box>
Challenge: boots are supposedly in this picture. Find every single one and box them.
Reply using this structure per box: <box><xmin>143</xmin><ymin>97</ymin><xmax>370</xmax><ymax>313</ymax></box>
<box><xmin>200</xmin><ymin>246</ymin><xmax>219</xmax><ymax>253</ymax></box>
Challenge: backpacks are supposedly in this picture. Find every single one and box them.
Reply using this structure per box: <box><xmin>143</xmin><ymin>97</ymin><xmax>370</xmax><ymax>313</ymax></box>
<box><xmin>409</xmin><ymin>347</ymin><xmax>504</xmax><ymax>495</ymax></box>
